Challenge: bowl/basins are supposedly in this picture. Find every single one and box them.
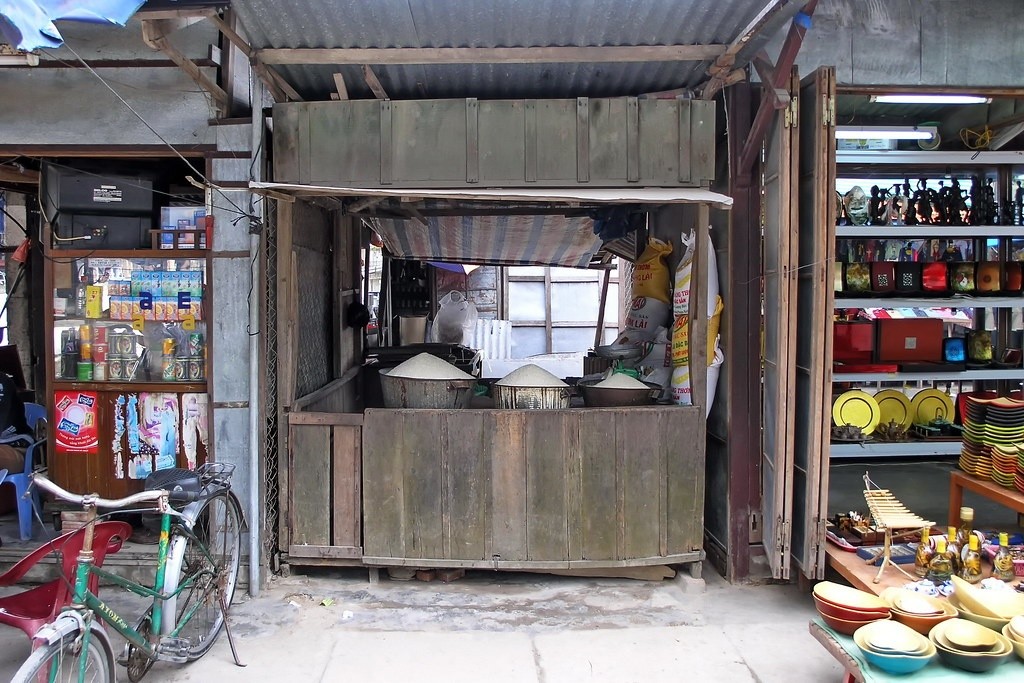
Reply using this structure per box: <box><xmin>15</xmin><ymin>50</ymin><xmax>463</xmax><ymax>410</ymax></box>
<box><xmin>812</xmin><ymin>574</ymin><xmax>1024</xmax><ymax>675</ymax></box>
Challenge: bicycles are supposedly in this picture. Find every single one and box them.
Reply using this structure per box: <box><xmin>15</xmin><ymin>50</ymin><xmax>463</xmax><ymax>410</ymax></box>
<box><xmin>0</xmin><ymin>462</ymin><xmax>251</xmax><ymax>683</ymax></box>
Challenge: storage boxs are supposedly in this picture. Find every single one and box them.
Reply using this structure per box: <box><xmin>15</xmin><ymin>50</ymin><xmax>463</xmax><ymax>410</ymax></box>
<box><xmin>87</xmin><ymin>286</ymin><xmax>102</xmax><ymax>318</ymax></box>
<box><xmin>837</xmin><ymin>139</ymin><xmax>898</xmax><ymax>149</ymax></box>
<box><xmin>833</xmin><ymin>318</ymin><xmax>965</xmax><ymax>373</ymax></box>
<box><xmin>108</xmin><ymin>269</ymin><xmax>202</xmax><ymax>321</ymax></box>
<box><xmin>161</xmin><ymin>206</ymin><xmax>207</xmax><ymax>249</ymax></box>
<box><xmin>857</xmin><ymin>543</ymin><xmax>918</xmax><ymax>568</ymax></box>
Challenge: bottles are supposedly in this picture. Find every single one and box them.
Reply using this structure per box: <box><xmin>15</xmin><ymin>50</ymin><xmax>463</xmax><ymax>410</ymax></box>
<box><xmin>75</xmin><ymin>276</ymin><xmax>90</xmax><ymax>317</ymax></box>
<box><xmin>392</xmin><ymin>276</ymin><xmax>432</xmax><ymax>309</ymax></box>
<box><xmin>914</xmin><ymin>506</ymin><xmax>1014</xmax><ymax>586</ymax></box>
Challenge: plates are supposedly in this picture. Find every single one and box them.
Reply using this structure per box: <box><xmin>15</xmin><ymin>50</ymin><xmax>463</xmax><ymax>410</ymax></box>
<box><xmin>911</xmin><ymin>389</ymin><xmax>955</xmax><ymax>427</ymax></box>
<box><xmin>958</xmin><ymin>389</ymin><xmax>1024</xmax><ymax>494</ymax></box>
<box><xmin>833</xmin><ymin>390</ymin><xmax>881</xmax><ymax>436</ymax></box>
<box><xmin>872</xmin><ymin>389</ymin><xmax>914</xmax><ymax>434</ymax></box>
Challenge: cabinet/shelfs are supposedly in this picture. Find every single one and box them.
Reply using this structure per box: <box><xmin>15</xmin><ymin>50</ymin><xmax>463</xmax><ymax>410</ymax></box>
<box><xmin>830</xmin><ymin>149</ymin><xmax>1023</xmax><ymax>457</ymax></box>
<box><xmin>392</xmin><ymin>279</ymin><xmax>432</xmax><ymax>318</ymax></box>
<box><xmin>43</xmin><ymin>248</ymin><xmax>210</xmax><ymax>531</ymax></box>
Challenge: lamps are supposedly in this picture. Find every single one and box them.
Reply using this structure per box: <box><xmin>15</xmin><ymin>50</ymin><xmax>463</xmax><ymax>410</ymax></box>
<box><xmin>834</xmin><ymin>126</ymin><xmax>936</xmax><ymax>140</ymax></box>
<box><xmin>867</xmin><ymin>95</ymin><xmax>992</xmax><ymax>104</ymax></box>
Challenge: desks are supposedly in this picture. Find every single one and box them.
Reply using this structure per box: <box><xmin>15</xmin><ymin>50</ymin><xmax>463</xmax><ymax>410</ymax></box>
<box><xmin>949</xmin><ymin>470</ymin><xmax>1024</xmax><ymax>534</ymax></box>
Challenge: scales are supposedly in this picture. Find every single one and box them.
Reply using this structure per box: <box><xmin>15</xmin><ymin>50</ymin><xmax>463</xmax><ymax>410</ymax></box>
<box><xmin>596</xmin><ymin>345</ymin><xmax>643</xmax><ymax>378</ymax></box>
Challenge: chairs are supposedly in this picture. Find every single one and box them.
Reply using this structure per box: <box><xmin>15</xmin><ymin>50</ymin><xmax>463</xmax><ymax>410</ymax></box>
<box><xmin>0</xmin><ymin>401</ymin><xmax>48</xmax><ymax>541</ymax></box>
<box><xmin>1</xmin><ymin>521</ymin><xmax>131</xmax><ymax>683</ymax></box>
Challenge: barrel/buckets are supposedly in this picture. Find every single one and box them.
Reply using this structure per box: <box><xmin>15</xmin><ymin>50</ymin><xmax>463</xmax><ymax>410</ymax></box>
<box><xmin>379</xmin><ymin>367</ymin><xmax>478</xmax><ymax>409</ymax></box>
<box><xmin>393</xmin><ymin>312</ymin><xmax>430</xmax><ymax>346</ymax></box>
<box><xmin>491</xmin><ymin>381</ymin><xmax>572</xmax><ymax>409</ymax></box>
<box><xmin>578</xmin><ymin>379</ymin><xmax>664</xmax><ymax>407</ymax></box>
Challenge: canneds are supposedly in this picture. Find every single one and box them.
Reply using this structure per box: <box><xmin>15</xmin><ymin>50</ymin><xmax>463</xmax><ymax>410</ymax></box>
<box><xmin>162</xmin><ymin>332</ymin><xmax>204</xmax><ymax>381</ymax></box>
<box><xmin>76</xmin><ymin>324</ymin><xmax>137</xmax><ymax>381</ymax></box>
